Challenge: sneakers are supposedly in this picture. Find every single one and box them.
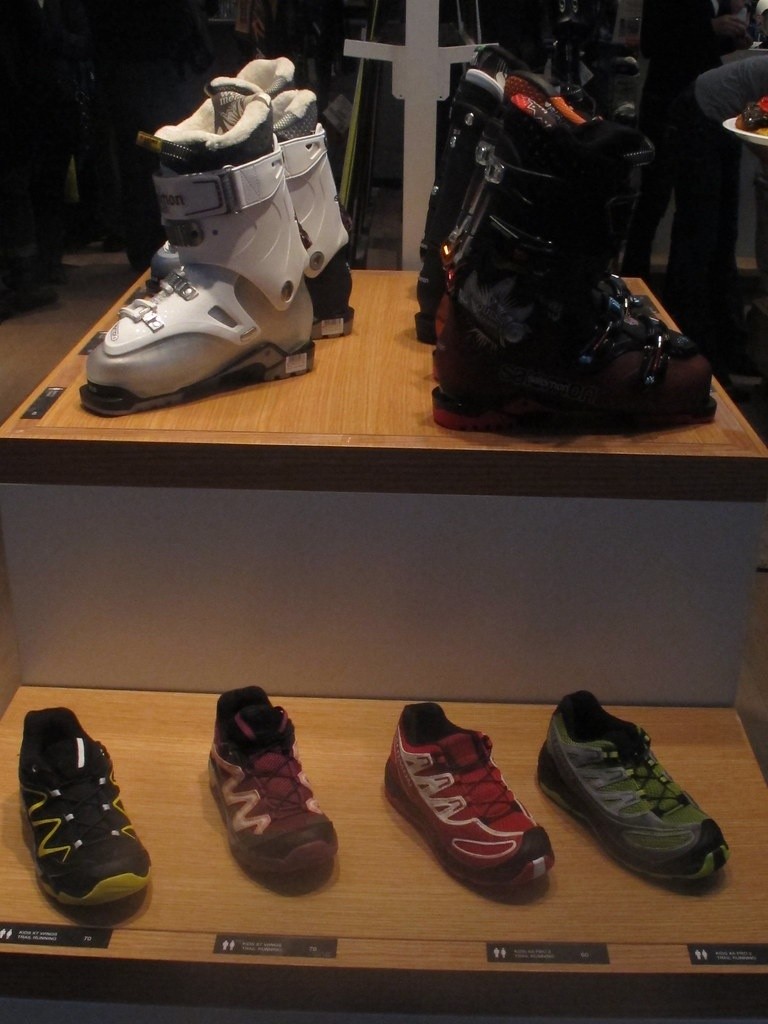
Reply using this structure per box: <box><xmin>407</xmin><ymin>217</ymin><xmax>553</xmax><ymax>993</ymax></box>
<box><xmin>207</xmin><ymin>685</ymin><xmax>340</xmax><ymax>876</ymax></box>
<box><xmin>383</xmin><ymin>702</ymin><xmax>555</xmax><ymax>887</ymax></box>
<box><xmin>18</xmin><ymin>708</ymin><xmax>153</xmax><ymax>906</ymax></box>
<box><xmin>537</xmin><ymin>688</ymin><xmax>732</xmax><ymax>881</ymax></box>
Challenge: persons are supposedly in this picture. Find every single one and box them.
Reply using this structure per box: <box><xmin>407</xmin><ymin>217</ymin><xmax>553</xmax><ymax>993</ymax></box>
<box><xmin>0</xmin><ymin>0</ymin><xmax>767</xmax><ymax>369</ymax></box>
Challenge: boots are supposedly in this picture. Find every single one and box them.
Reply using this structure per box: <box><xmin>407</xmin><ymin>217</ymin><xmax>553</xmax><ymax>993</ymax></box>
<box><xmin>270</xmin><ymin>86</ymin><xmax>354</xmax><ymax>340</ymax></box>
<box><xmin>80</xmin><ymin>74</ymin><xmax>318</xmax><ymax>413</ymax></box>
<box><xmin>412</xmin><ymin>69</ymin><xmax>597</xmax><ymax>345</ymax></box>
<box><xmin>428</xmin><ymin>65</ymin><xmax>712</xmax><ymax>434</ymax></box>
